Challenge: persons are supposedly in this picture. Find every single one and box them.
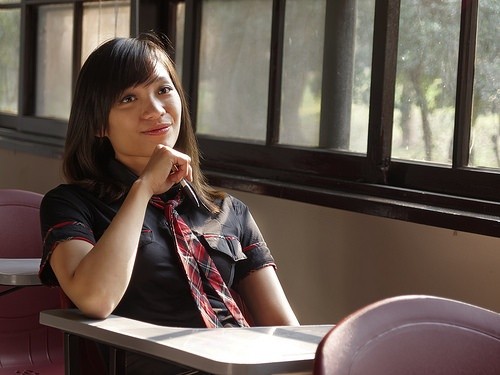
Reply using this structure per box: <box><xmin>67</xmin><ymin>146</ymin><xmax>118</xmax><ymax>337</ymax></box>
<box><xmin>38</xmin><ymin>37</ymin><xmax>301</xmax><ymax>375</ymax></box>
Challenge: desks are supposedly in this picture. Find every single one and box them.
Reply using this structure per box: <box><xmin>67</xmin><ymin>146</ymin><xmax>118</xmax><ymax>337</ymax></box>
<box><xmin>37</xmin><ymin>308</ymin><xmax>335</xmax><ymax>375</ymax></box>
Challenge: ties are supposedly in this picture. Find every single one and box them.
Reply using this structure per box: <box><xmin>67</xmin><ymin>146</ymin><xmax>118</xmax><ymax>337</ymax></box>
<box><xmin>148</xmin><ymin>191</ymin><xmax>250</xmax><ymax>330</ymax></box>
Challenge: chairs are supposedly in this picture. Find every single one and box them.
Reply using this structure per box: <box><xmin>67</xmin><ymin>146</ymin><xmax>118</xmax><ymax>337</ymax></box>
<box><xmin>315</xmin><ymin>294</ymin><xmax>500</xmax><ymax>375</ymax></box>
<box><xmin>0</xmin><ymin>189</ymin><xmax>67</xmax><ymax>375</ymax></box>
